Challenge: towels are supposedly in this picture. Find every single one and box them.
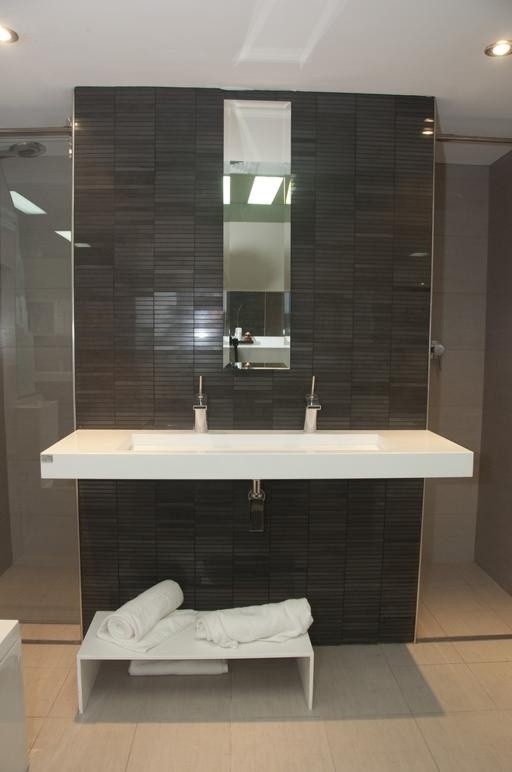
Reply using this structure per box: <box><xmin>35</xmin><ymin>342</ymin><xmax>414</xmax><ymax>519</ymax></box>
<box><xmin>98</xmin><ymin>578</ymin><xmax>314</xmax><ymax>651</ymax></box>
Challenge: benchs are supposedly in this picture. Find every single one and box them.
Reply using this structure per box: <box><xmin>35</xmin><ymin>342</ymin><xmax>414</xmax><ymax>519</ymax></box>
<box><xmin>75</xmin><ymin>610</ymin><xmax>315</xmax><ymax>712</ymax></box>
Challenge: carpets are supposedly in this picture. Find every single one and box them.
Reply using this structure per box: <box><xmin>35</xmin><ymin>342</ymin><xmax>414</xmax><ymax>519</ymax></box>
<box><xmin>75</xmin><ymin>642</ymin><xmax>444</xmax><ymax>722</ymax></box>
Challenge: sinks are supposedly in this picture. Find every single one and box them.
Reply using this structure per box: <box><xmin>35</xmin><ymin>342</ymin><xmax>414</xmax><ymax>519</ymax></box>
<box><xmin>122</xmin><ymin>432</ymin><xmax>383</xmax><ymax>452</ymax></box>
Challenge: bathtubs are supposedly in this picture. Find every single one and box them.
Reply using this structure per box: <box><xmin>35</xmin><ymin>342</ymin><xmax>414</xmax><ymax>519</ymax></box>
<box><xmin>228</xmin><ymin>334</ymin><xmax>290</xmax><ymax>366</ymax></box>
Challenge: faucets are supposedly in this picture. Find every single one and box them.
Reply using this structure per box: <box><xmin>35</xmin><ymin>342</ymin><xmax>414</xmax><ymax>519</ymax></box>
<box><xmin>301</xmin><ymin>374</ymin><xmax>321</xmax><ymax>431</ymax></box>
<box><xmin>282</xmin><ymin>329</ymin><xmax>288</xmax><ymax>347</ymax></box>
<box><xmin>192</xmin><ymin>374</ymin><xmax>209</xmax><ymax>432</ymax></box>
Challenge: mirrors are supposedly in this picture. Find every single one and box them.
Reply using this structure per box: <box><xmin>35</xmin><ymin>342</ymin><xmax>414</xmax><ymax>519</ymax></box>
<box><xmin>223</xmin><ymin>172</ymin><xmax>292</xmax><ymax>371</ymax></box>
